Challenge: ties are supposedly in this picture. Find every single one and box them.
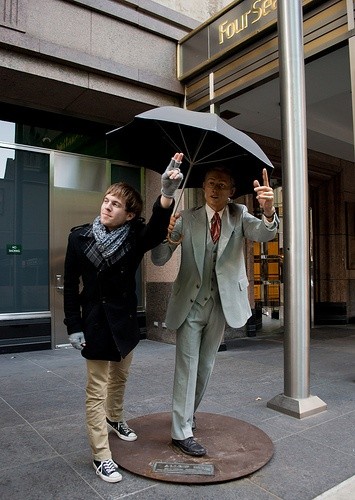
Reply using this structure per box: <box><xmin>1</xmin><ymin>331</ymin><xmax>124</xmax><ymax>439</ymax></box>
<box><xmin>209</xmin><ymin>213</ymin><xmax>222</xmax><ymax>245</ymax></box>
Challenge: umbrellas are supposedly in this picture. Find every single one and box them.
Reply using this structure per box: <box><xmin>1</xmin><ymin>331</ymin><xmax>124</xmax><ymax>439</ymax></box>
<box><xmin>106</xmin><ymin>105</ymin><xmax>276</xmax><ymax>246</ymax></box>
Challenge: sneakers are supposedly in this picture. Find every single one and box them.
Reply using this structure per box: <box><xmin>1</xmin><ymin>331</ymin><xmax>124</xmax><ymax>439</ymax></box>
<box><xmin>106</xmin><ymin>418</ymin><xmax>138</xmax><ymax>441</ymax></box>
<box><xmin>92</xmin><ymin>460</ymin><xmax>122</xmax><ymax>482</ymax></box>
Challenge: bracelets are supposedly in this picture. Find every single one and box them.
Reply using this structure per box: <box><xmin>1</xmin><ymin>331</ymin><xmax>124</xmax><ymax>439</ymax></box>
<box><xmin>263</xmin><ymin>210</ymin><xmax>275</xmax><ymax>219</ymax></box>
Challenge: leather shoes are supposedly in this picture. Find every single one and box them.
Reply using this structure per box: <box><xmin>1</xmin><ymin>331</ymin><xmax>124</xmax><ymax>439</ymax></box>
<box><xmin>172</xmin><ymin>437</ymin><xmax>206</xmax><ymax>455</ymax></box>
<box><xmin>191</xmin><ymin>416</ymin><xmax>196</xmax><ymax>428</ymax></box>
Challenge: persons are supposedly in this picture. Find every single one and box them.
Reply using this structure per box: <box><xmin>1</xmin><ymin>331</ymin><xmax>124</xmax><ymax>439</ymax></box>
<box><xmin>150</xmin><ymin>167</ymin><xmax>281</xmax><ymax>457</ymax></box>
<box><xmin>63</xmin><ymin>152</ymin><xmax>183</xmax><ymax>483</ymax></box>
